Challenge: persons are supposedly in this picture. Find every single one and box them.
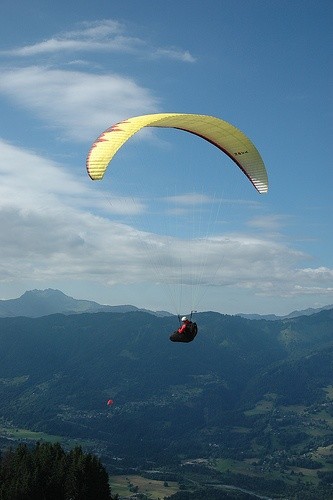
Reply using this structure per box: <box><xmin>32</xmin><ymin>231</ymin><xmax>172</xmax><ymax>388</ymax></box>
<box><xmin>178</xmin><ymin>316</ymin><xmax>193</xmax><ymax>334</ymax></box>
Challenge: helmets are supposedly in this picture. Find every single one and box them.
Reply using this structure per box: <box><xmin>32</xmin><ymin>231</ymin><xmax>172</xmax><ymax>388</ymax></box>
<box><xmin>181</xmin><ymin>317</ymin><xmax>188</xmax><ymax>322</ymax></box>
